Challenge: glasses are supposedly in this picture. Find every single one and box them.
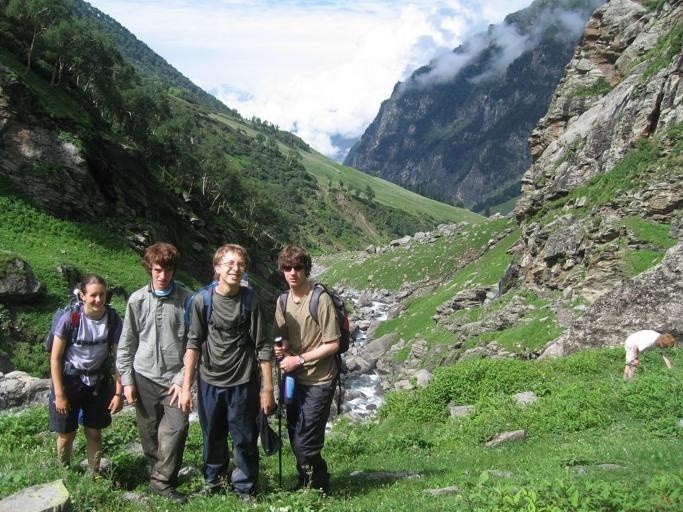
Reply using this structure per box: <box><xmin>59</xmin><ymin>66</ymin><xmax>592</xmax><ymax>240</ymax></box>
<box><xmin>215</xmin><ymin>261</ymin><xmax>246</xmax><ymax>269</ymax></box>
<box><xmin>283</xmin><ymin>263</ymin><xmax>306</xmax><ymax>272</ymax></box>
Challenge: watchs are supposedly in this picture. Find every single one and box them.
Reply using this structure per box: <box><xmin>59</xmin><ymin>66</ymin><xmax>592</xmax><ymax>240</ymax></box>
<box><xmin>298</xmin><ymin>354</ymin><xmax>305</xmax><ymax>368</ymax></box>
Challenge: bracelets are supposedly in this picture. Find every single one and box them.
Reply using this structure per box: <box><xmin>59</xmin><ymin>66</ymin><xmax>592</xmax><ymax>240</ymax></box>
<box><xmin>115</xmin><ymin>390</ymin><xmax>124</xmax><ymax>399</ymax></box>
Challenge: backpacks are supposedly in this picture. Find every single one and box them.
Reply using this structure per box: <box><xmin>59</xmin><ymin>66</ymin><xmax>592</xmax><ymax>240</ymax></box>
<box><xmin>185</xmin><ymin>282</ymin><xmax>253</xmax><ymax>370</ymax></box>
<box><xmin>45</xmin><ymin>283</ymin><xmax>116</xmax><ymax>376</ymax></box>
<box><xmin>280</xmin><ymin>283</ymin><xmax>349</xmax><ymax>416</ymax></box>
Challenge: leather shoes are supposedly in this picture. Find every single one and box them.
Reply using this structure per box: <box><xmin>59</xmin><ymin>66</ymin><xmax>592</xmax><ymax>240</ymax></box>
<box><xmin>146</xmin><ymin>478</ymin><xmax>329</xmax><ymax>504</ymax></box>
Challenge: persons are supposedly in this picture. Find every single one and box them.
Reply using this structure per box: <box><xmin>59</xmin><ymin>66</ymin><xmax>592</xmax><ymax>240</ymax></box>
<box><xmin>116</xmin><ymin>240</ymin><xmax>194</xmax><ymax>504</ymax></box>
<box><xmin>48</xmin><ymin>274</ymin><xmax>123</xmax><ymax>491</ymax></box>
<box><xmin>179</xmin><ymin>242</ymin><xmax>275</xmax><ymax>502</ymax></box>
<box><xmin>623</xmin><ymin>329</ymin><xmax>675</xmax><ymax>382</ymax></box>
<box><xmin>269</xmin><ymin>243</ymin><xmax>342</xmax><ymax>492</ymax></box>
